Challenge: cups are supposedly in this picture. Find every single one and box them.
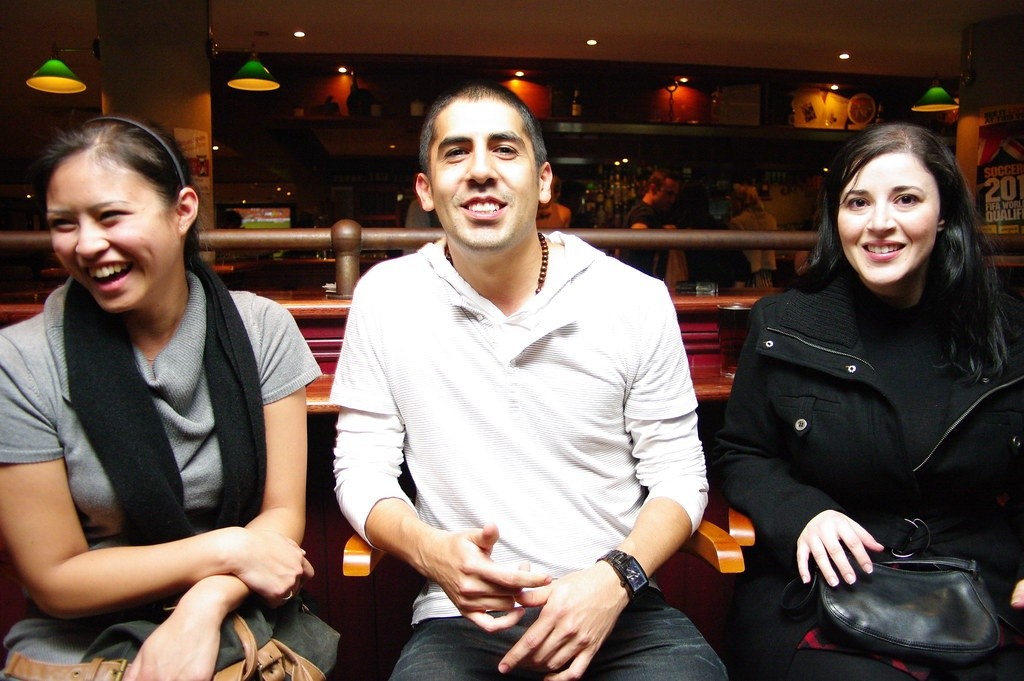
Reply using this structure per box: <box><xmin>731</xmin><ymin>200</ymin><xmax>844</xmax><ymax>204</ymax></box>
<box><xmin>411</xmin><ymin>98</ymin><xmax>430</xmax><ymax>117</ymax></box>
<box><xmin>718</xmin><ymin>301</ymin><xmax>755</xmax><ymax>379</ymax></box>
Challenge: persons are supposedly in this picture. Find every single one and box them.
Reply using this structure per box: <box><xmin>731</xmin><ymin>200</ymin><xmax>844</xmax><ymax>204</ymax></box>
<box><xmin>704</xmin><ymin>126</ymin><xmax>1024</xmax><ymax>681</ymax></box>
<box><xmin>216</xmin><ymin>169</ymin><xmax>822</xmax><ymax>291</ymax></box>
<box><xmin>0</xmin><ymin>115</ymin><xmax>322</xmax><ymax>681</ymax></box>
<box><xmin>330</xmin><ymin>73</ymin><xmax>729</xmax><ymax>681</ymax></box>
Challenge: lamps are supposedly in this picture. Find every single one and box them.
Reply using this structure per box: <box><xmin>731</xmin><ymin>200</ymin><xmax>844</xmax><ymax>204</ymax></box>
<box><xmin>911</xmin><ymin>67</ymin><xmax>977</xmax><ymax>112</ymax></box>
<box><xmin>208</xmin><ymin>38</ymin><xmax>280</xmax><ymax>91</ymax></box>
<box><xmin>26</xmin><ymin>38</ymin><xmax>101</xmax><ymax>94</ymax></box>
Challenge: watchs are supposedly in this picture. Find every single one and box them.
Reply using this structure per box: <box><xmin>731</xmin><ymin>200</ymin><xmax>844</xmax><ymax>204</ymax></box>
<box><xmin>595</xmin><ymin>550</ymin><xmax>649</xmax><ymax>601</ymax></box>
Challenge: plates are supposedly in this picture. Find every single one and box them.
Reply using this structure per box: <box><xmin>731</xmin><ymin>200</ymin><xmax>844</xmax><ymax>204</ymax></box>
<box><xmin>846</xmin><ymin>93</ymin><xmax>876</xmax><ymax>125</ymax></box>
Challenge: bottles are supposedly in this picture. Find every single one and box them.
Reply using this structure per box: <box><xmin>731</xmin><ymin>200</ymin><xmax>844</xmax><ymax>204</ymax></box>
<box><xmin>681</xmin><ymin>167</ymin><xmax>824</xmax><ymax>221</ymax></box>
<box><xmin>570</xmin><ymin>82</ymin><xmax>583</xmax><ymax>121</ymax></box>
<box><xmin>709</xmin><ymin>86</ymin><xmax>720</xmax><ymax>125</ymax></box>
<box><xmin>875</xmin><ymin>103</ymin><xmax>885</xmax><ymax>125</ymax></box>
<box><xmin>311</xmin><ymin>166</ymin><xmax>414</xmax><ymax>185</ymax></box>
<box><xmin>585</xmin><ymin>159</ymin><xmax>656</xmax><ymax>227</ymax></box>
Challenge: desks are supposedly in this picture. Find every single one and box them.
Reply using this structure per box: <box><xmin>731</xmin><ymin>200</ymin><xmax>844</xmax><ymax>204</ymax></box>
<box><xmin>307</xmin><ymin>369</ymin><xmax>733</xmax><ymax>413</ymax></box>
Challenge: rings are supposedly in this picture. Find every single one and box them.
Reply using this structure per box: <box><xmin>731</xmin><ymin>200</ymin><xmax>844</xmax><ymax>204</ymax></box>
<box><xmin>281</xmin><ymin>590</ymin><xmax>294</xmax><ymax>601</ymax></box>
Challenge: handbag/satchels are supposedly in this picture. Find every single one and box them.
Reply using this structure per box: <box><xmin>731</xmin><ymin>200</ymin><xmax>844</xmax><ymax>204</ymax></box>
<box><xmin>777</xmin><ymin>553</ymin><xmax>1005</xmax><ymax>664</ymax></box>
<box><xmin>10</xmin><ymin>587</ymin><xmax>340</xmax><ymax>681</ymax></box>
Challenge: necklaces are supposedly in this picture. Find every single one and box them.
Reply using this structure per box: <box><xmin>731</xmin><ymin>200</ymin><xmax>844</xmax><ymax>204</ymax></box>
<box><xmin>445</xmin><ymin>230</ymin><xmax>548</xmax><ymax>296</ymax></box>
